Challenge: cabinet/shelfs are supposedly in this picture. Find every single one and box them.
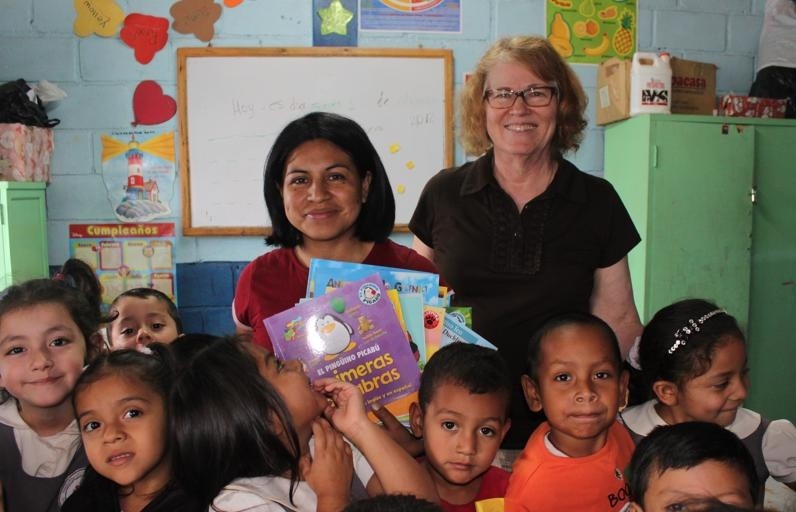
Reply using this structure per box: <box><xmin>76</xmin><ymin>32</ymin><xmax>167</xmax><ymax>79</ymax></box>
<box><xmin>1</xmin><ymin>180</ymin><xmax>49</xmax><ymax>294</ymax></box>
<box><xmin>604</xmin><ymin>114</ymin><xmax>796</xmax><ymax>425</ymax></box>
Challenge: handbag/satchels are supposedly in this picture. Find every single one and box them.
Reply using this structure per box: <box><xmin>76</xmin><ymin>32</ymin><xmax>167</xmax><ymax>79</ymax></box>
<box><xmin>0</xmin><ymin>78</ymin><xmax>60</xmax><ymax>128</ymax></box>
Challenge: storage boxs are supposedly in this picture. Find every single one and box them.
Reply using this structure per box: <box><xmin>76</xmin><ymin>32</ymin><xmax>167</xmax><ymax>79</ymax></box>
<box><xmin>596</xmin><ymin>55</ymin><xmax>718</xmax><ymax>125</ymax></box>
<box><xmin>0</xmin><ymin>122</ymin><xmax>53</xmax><ymax>186</ymax></box>
<box><xmin>717</xmin><ymin>94</ymin><xmax>792</xmax><ymax>118</ymax></box>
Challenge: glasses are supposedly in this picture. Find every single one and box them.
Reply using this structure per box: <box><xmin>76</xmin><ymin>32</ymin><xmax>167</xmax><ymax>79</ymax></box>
<box><xmin>482</xmin><ymin>86</ymin><xmax>557</xmax><ymax>109</ymax></box>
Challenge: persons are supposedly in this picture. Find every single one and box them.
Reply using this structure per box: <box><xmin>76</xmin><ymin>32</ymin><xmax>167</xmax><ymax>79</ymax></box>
<box><xmin>626</xmin><ymin>420</ymin><xmax>760</xmax><ymax>511</ymax></box>
<box><xmin>505</xmin><ymin>312</ymin><xmax>636</xmax><ymax>511</ymax></box>
<box><xmin>409</xmin><ymin>36</ymin><xmax>645</xmax><ymax>365</ymax></box>
<box><xmin>616</xmin><ymin>299</ymin><xmax>796</xmax><ymax>512</ymax></box>
<box><xmin>0</xmin><ymin>255</ymin><xmax>517</xmax><ymax>511</ymax></box>
<box><xmin>232</xmin><ymin>111</ymin><xmax>455</xmax><ymax>362</ymax></box>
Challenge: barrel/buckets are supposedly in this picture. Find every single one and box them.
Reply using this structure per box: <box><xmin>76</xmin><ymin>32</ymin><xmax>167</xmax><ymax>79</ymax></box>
<box><xmin>629</xmin><ymin>52</ymin><xmax>673</xmax><ymax>117</ymax></box>
